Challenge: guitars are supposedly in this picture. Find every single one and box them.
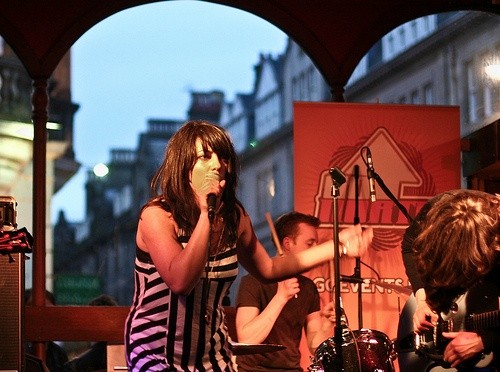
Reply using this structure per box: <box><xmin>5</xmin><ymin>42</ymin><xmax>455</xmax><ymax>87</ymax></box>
<box><xmin>396</xmin><ymin>287</ymin><xmax>500</xmax><ymax>372</ymax></box>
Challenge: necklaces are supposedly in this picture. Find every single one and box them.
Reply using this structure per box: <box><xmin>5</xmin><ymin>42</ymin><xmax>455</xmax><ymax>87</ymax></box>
<box><xmin>203</xmin><ymin>228</ymin><xmax>225</xmax><ymax>325</ymax></box>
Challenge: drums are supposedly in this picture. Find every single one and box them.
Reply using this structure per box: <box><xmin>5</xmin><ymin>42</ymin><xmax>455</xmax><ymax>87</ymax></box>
<box><xmin>307</xmin><ymin>328</ymin><xmax>398</xmax><ymax>372</ymax></box>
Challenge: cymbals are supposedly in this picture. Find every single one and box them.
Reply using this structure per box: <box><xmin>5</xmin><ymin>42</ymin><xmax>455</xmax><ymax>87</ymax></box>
<box><xmin>231</xmin><ymin>343</ymin><xmax>288</xmax><ymax>356</ymax></box>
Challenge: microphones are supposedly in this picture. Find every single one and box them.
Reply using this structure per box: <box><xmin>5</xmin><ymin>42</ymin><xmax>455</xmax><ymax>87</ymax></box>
<box><xmin>366</xmin><ymin>148</ymin><xmax>376</xmax><ymax>202</ymax></box>
<box><xmin>207</xmin><ymin>173</ymin><xmax>222</xmax><ymax>222</ymax></box>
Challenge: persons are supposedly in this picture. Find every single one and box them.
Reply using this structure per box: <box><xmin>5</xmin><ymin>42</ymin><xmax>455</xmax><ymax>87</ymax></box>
<box><xmin>64</xmin><ymin>296</ymin><xmax>118</xmax><ymax>372</ymax></box>
<box><xmin>402</xmin><ymin>189</ymin><xmax>500</xmax><ymax>372</ymax></box>
<box><xmin>235</xmin><ymin>212</ymin><xmax>335</xmax><ymax>372</ymax></box>
<box><xmin>22</xmin><ymin>289</ymin><xmax>68</xmax><ymax>372</ymax></box>
<box><xmin>124</xmin><ymin>121</ymin><xmax>375</xmax><ymax>372</ymax></box>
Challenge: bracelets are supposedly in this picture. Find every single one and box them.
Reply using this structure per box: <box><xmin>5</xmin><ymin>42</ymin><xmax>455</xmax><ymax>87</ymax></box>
<box><xmin>338</xmin><ymin>240</ymin><xmax>347</xmax><ymax>257</ymax></box>
